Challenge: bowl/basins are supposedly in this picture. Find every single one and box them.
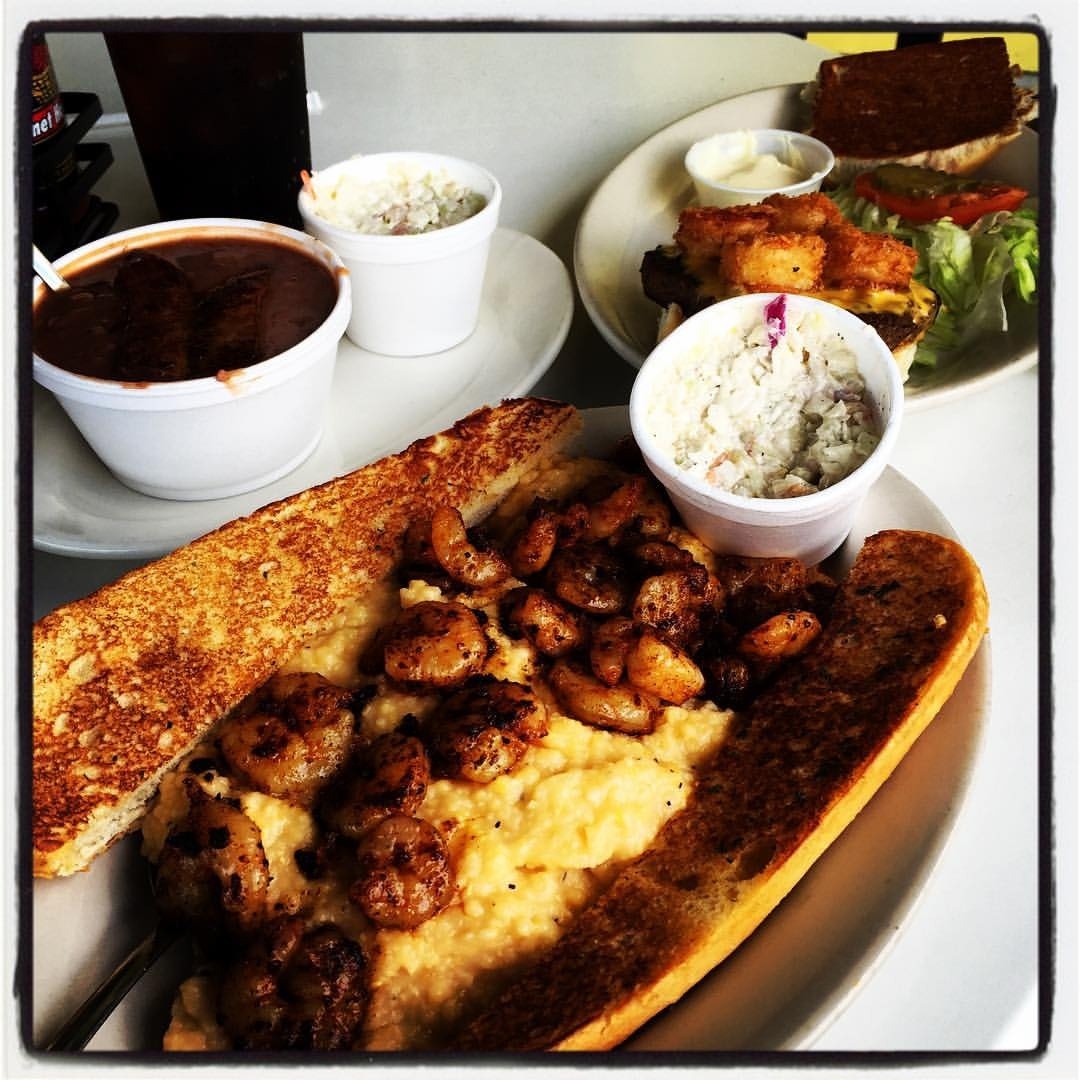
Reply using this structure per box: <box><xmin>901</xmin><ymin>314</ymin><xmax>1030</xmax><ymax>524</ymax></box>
<box><xmin>631</xmin><ymin>293</ymin><xmax>904</xmax><ymax>568</ymax></box>
<box><xmin>296</xmin><ymin>152</ymin><xmax>501</xmax><ymax>359</ymax></box>
<box><xmin>682</xmin><ymin>129</ymin><xmax>837</xmax><ymax>209</ymax></box>
<box><xmin>31</xmin><ymin>219</ymin><xmax>355</xmax><ymax>502</ymax></box>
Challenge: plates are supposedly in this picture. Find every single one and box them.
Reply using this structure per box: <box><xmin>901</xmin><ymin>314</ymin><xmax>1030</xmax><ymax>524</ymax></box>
<box><xmin>31</xmin><ymin>224</ymin><xmax>574</xmax><ymax>560</ymax></box>
<box><xmin>571</xmin><ymin>81</ymin><xmax>1039</xmax><ymax>406</ymax></box>
<box><xmin>32</xmin><ymin>404</ymin><xmax>961</xmax><ymax>1052</ymax></box>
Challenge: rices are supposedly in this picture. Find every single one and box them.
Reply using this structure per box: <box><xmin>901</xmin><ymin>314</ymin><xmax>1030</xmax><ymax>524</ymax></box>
<box><xmin>312</xmin><ymin>154</ymin><xmax>488</xmax><ymax>235</ymax></box>
<box><xmin>660</xmin><ymin>312</ymin><xmax>850</xmax><ymax>490</ymax></box>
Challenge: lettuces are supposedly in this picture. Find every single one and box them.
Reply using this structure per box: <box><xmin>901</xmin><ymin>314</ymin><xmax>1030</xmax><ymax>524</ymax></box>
<box><xmin>823</xmin><ymin>162</ymin><xmax>1038</xmax><ymax>393</ymax></box>
